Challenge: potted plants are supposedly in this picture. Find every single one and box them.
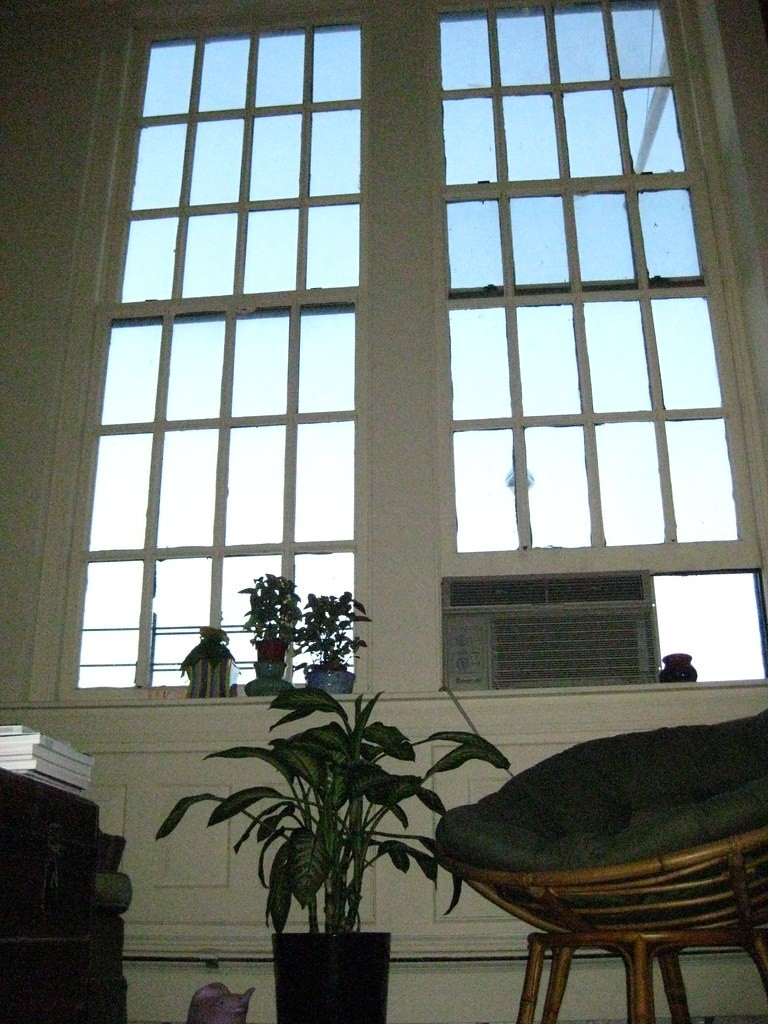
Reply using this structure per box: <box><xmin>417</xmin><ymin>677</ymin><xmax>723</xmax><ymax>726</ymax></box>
<box><xmin>239</xmin><ymin>574</ymin><xmax>301</xmax><ymax>662</ymax></box>
<box><xmin>154</xmin><ymin>687</ymin><xmax>508</xmax><ymax>1024</ymax></box>
<box><xmin>301</xmin><ymin>592</ymin><xmax>371</xmax><ymax>693</ymax></box>
<box><xmin>180</xmin><ymin>627</ymin><xmax>238</xmax><ymax>698</ymax></box>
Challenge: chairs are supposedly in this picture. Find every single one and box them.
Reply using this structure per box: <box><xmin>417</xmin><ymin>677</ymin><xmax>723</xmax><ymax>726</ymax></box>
<box><xmin>435</xmin><ymin>708</ymin><xmax>768</xmax><ymax>1024</ymax></box>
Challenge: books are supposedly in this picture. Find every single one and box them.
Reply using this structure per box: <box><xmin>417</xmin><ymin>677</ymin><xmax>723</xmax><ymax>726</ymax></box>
<box><xmin>0</xmin><ymin>732</ymin><xmax>95</xmax><ymax>797</ymax></box>
<box><xmin>0</xmin><ymin>725</ymin><xmax>32</xmax><ymax>737</ymax></box>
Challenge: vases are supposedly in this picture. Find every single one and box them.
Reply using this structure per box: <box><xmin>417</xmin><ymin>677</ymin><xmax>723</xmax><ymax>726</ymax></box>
<box><xmin>659</xmin><ymin>653</ymin><xmax>697</xmax><ymax>683</ymax></box>
<box><xmin>244</xmin><ymin>662</ymin><xmax>295</xmax><ymax>696</ymax></box>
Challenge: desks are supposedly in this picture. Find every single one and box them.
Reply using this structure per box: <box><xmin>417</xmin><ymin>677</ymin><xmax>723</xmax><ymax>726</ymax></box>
<box><xmin>0</xmin><ymin>765</ymin><xmax>99</xmax><ymax>1024</ymax></box>
